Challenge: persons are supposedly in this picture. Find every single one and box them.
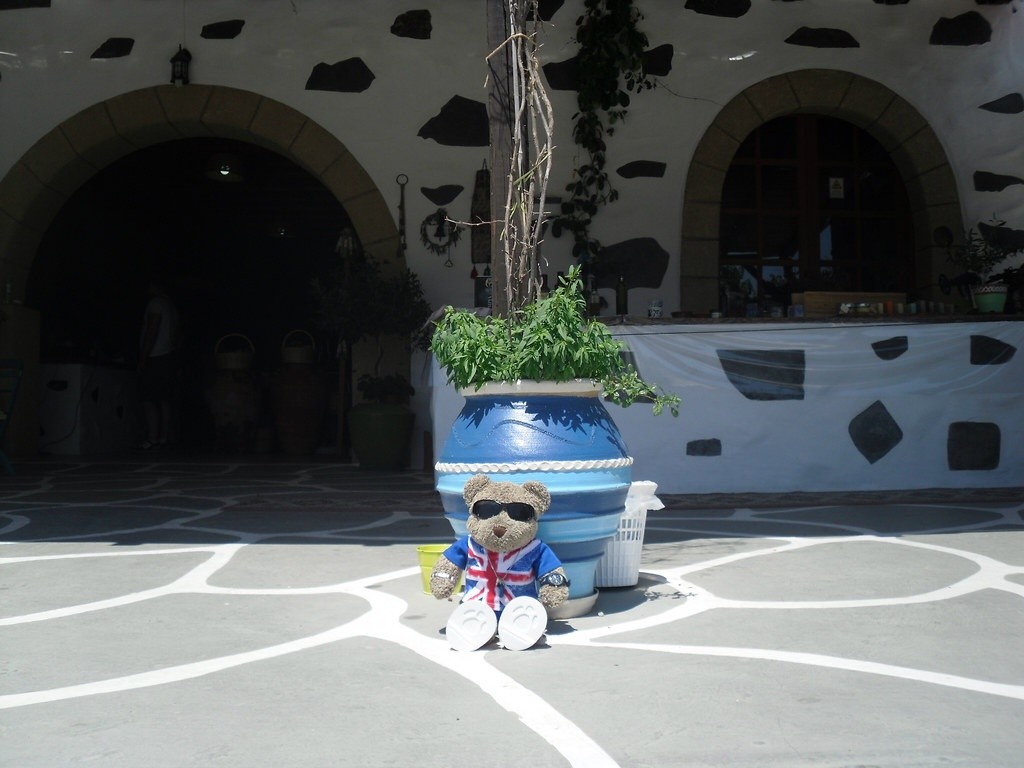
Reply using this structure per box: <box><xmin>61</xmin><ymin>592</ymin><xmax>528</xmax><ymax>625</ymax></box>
<box><xmin>141</xmin><ymin>276</ymin><xmax>180</xmax><ymax>450</ymax></box>
<box><xmin>933</xmin><ymin>225</ymin><xmax>970</xmax><ymax>300</ymax></box>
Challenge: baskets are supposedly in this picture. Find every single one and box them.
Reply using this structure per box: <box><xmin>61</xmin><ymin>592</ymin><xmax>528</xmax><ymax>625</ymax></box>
<box><xmin>215</xmin><ymin>334</ymin><xmax>255</xmax><ymax>368</ymax></box>
<box><xmin>281</xmin><ymin>330</ymin><xmax>315</xmax><ymax>363</ymax></box>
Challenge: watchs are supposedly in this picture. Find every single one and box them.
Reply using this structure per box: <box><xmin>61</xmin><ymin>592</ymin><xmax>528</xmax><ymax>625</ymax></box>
<box><xmin>539</xmin><ymin>571</ymin><xmax>568</xmax><ymax>588</ymax></box>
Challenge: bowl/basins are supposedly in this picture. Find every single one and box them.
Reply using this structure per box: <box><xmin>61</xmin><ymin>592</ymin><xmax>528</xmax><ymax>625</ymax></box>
<box><xmin>836</xmin><ymin>303</ymin><xmax>870</xmax><ymax>312</ymax></box>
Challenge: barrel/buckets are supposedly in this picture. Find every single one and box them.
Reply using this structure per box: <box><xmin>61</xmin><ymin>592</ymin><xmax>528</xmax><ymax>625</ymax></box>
<box><xmin>416</xmin><ymin>543</ymin><xmax>463</xmax><ymax>594</ymax></box>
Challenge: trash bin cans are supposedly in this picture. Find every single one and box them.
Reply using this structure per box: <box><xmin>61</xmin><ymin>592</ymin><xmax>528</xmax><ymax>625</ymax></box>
<box><xmin>593</xmin><ymin>480</ymin><xmax>658</xmax><ymax>588</ymax></box>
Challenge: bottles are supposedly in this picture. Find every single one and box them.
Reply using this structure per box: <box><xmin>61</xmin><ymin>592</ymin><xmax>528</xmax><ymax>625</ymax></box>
<box><xmin>541</xmin><ymin>270</ymin><xmax>628</xmax><ymax>317</ymax></box>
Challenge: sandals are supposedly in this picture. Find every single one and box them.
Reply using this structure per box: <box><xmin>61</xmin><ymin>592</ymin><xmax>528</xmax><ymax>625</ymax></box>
<box><xmin>130</xmin><ymin>439</ymin><xmax>161</xmax><ymax>450</ymax></box>
<box><xmin>160</xmin><ymin>442</ymin><xmax>171</xmax><ymax>450</ymax></box>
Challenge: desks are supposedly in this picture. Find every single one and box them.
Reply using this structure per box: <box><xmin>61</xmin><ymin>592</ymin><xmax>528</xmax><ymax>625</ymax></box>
<box><xmin>429</xmin><ymin>317</ymin><xmax>1024</xmax><ymax>511</ymax></box>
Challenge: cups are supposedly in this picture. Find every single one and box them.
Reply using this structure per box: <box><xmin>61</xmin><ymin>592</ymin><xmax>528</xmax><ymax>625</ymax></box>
<box><xmin>872</xmin><ymin>301</ymin><xmax>954</xmax><ymax>316</ymax></box>
<box><xmin>712</xmin><ymin>312</ymin><xmax>722</xmax><ymax>318</ymax></box>
<box><xmin>743</xmin><ymin>302</ymin><xmax>805</xmax><ymax>318</ymax></box>
<box><xmin>648</xmin><ymin>307</ymin><xmax>662</xmax><ymax>318</ymax></box>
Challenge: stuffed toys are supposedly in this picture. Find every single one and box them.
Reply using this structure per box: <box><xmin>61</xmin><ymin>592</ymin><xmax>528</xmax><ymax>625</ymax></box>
<box><xmin>430</xmin><ymin>474</ymin><xmax>570</xmax><ymax>651</ymax></box>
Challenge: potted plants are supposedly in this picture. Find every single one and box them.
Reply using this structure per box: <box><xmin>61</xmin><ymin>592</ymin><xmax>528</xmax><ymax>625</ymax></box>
<box><xmin>317</xmin><ymin>255</ymin><xmax>433</xmax><ymax>474</ymax></box>
<box><xmin>950</xmin><ymin>215</ymin><xmax>1012</xmax><ymax>313</ymax></box>
<box><xmin>428</xmin><ymin>274</ymin><xmax>682</xmax><ymax>622</ymax></box>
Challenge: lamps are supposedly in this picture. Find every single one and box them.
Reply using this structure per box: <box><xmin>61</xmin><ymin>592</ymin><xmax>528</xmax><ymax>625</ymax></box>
<box><xmin>217</xmin><ymin>160</ymin><xmax>233</xmax><ymax>177</ymax></box>
<box><xmin>170</xmin><ymin>42</ymin><xmax>189</xmax><ymax>87</ymax></box>
